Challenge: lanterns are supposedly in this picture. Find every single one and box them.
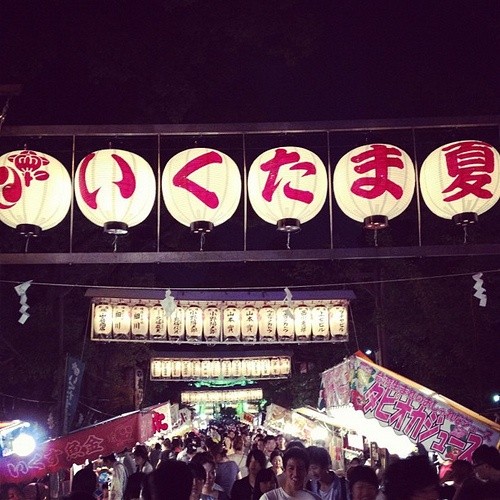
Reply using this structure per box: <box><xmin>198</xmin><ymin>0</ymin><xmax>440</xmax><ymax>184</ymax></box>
<box><xmin>148</xmin><ymin>358</ymin><xmax>292</xmax><ymax>379</ymax></box>
<box><xmin>247</xmin><ymin>145</ymin><xmax>331</xmax><ymax>232</ymax></box>
<box><xmin>419</xmin><ymin>139</ymin><xmax>500</xmax><ymax>226</ymax></box>
<box><xmin>159</xmin><ymin>148</ymin><xmax>244</xmax><ymax>234</ymax></box>
<box><xmin>96</xmin><ymin>301</ymin><xmax>349</xmax><ymax>343</ymax></box>
<box><xmin>180</xmin><ymin>388</ymin><xmax>262</xmax><ymax>401</ymax></box>
<box><xmin>72</xmin><ymin>148</ymin><xmax>156</xmax><ymax>239</ymax></box>
<box><xmin>0</xmin><ymin>150</ymin><xmax>74</xmax><ymax>237</ymax></box>
<box><xmin>332</xmin><ymin>143</ymin><xmax>416</xmax><ymax>230</ymax></box>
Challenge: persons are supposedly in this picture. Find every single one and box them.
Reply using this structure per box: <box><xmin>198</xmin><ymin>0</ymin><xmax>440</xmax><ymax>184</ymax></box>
<box><xmin>0</xmin><ymin>415</ymin><xmax>500</xmax><ymax>500</ymax></box>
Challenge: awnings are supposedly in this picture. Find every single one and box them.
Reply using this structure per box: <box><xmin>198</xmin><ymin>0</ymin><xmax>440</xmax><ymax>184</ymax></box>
<box><xmin>0</xmin><ymin>403</ymin><xmax>191</xmax><ymax>484</ymax></box>
<box><xmin>248</xmin><ymin>355</ymin><xmax>500</xmax><ymax>471</ymax></box>
